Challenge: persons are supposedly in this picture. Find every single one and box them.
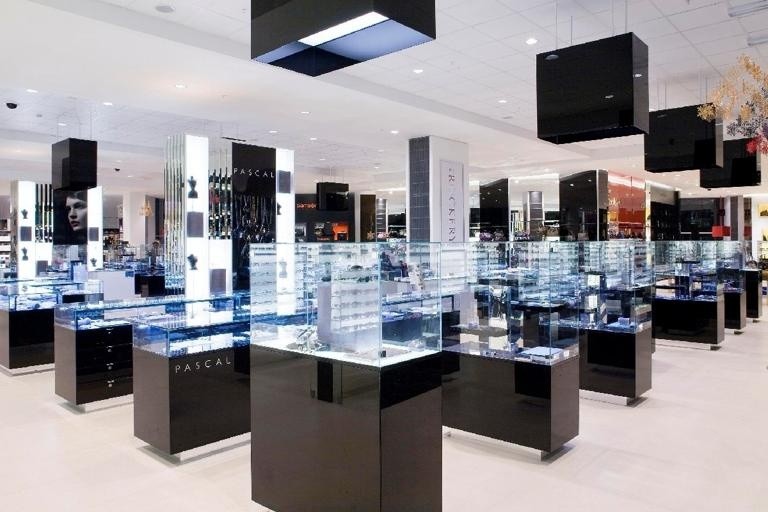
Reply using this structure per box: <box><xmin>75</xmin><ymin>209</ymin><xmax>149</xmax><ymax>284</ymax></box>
<box><xmin>65</xmin><ymin>190</ymin><xmax>87</xmax><ymax>231</ymax></box>
